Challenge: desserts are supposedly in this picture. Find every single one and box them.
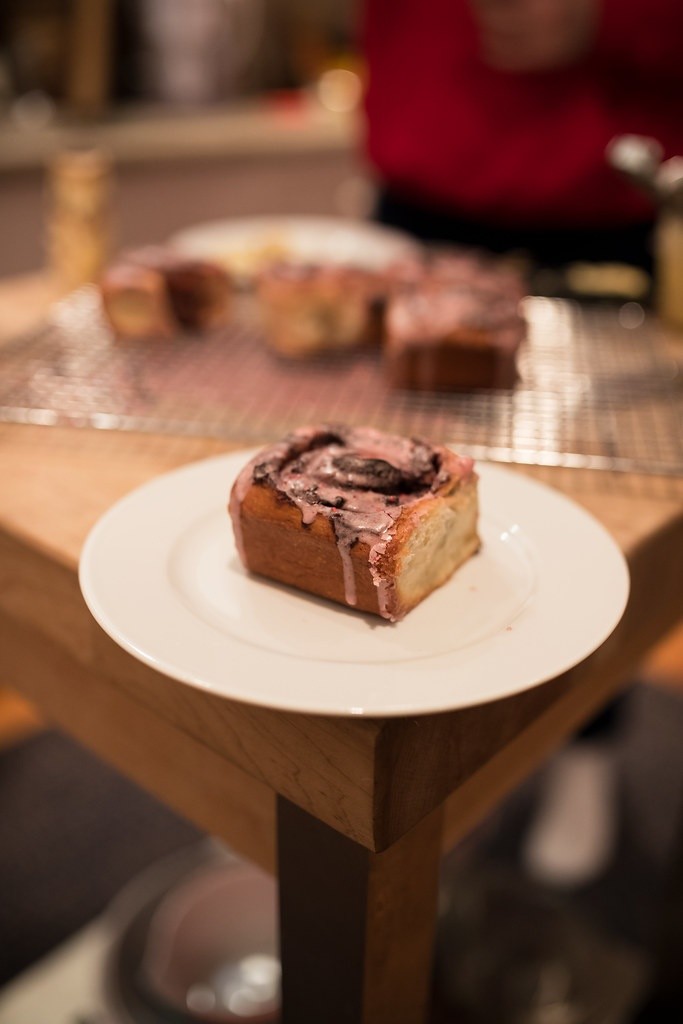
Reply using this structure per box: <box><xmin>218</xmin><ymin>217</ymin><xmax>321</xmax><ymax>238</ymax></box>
<box><xmin>227</xmin><ymin>419</ymin><xmax>482</xmax><ymax>622</ymax></box>
<box><xmin>101</xmin><ymin>241</ymin><xmax>528</xmax><ymax>392</ymax></box>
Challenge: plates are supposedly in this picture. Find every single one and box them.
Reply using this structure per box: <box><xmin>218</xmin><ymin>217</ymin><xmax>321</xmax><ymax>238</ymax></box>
<box><xmin>77</xmin><ymin>451</ymin><xmax>633</xmax><ymax>718</ymax></box>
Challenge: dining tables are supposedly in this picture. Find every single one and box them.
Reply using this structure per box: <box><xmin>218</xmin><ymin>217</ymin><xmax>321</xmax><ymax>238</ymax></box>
<box><xmin>0</xmin><ymin>295</ymin><xmax>683</xmax><ymax>1024</ymax></box>
